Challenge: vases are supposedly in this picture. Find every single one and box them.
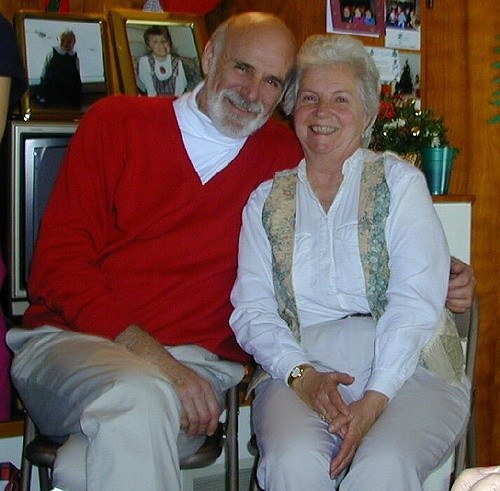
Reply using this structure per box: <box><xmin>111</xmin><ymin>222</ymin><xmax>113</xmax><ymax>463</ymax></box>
<box><xmin>423</xmin><ymin>145</ymin><xmax>456</xmax><ymax>197</ymax></box>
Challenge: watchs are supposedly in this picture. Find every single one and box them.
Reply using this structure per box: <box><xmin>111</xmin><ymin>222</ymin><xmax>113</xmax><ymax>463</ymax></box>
<box><xmin>288</xmin><ymin>364</ymin><xmax>314</xmax><ymax>388</ymax></box>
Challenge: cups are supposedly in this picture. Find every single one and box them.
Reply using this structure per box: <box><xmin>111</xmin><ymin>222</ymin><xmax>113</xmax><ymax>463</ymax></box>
<box><xmin>421</xmin><ymin>145</ymin><xmax>453</xmax><ymax>196</ymax></box>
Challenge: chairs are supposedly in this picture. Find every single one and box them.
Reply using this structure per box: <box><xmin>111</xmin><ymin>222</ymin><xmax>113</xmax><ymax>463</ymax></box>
<box><xmin>11</xmin><ymin>335</ymin><xmax>246</xmax><ymax>491</ymax></box>
<box><xmin>246</xmin><ymin>254</ymin><xmax>480</xmax><ymax>491</ymax></box>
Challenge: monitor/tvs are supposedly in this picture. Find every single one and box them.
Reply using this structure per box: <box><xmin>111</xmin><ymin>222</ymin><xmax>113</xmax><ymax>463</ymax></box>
<box><xmin>11</xmin><ymin>121</ymin><xmax>79</xmax><ymax>300</ymax></box>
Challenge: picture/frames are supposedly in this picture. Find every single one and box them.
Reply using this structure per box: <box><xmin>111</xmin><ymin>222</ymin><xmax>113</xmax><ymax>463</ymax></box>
<box><xmin>7</xmin><ymin>119</ymin><xmax>79</xmax><ymax>301</ymax></box>
<box><xmin>14</xmin><ymin>8</ymin><xmax>117</xmax><ymax>124</ymax></box>
<box><xmin>108</xmin><ymin>6</ymin><xmax>207</xmax><ymax>99</ymax></box>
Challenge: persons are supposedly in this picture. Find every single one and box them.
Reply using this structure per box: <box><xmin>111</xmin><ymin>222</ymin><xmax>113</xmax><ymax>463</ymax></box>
<box><xmin>40</xmin><ymin>29</ymin><xmax>83</xmax><ymax>110</ymax></box>
<box><xmin>229</xmin><ymin>33</ymin><xmax>470</xmax><ymax>491</ymax></box>
<box><xmin>6</xmin><ymin>11</ymin><xmax>477</xmax><ymax>491</ymax></box>
<box><xmin>136</xmin><ymin>25</ymin><xmax>187</xmax><ymax>98</ymax></box>
<box><xmin>343</xmin><ymin>6</ymin><xmax>420</xmax><ymax>30</ymax></box>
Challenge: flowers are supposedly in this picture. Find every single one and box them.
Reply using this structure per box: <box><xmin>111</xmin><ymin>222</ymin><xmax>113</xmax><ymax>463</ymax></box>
<box><xmin>370</xmin><ymin>82</ymin><xmax>450</xmax><ymax>157</ymax></box>
